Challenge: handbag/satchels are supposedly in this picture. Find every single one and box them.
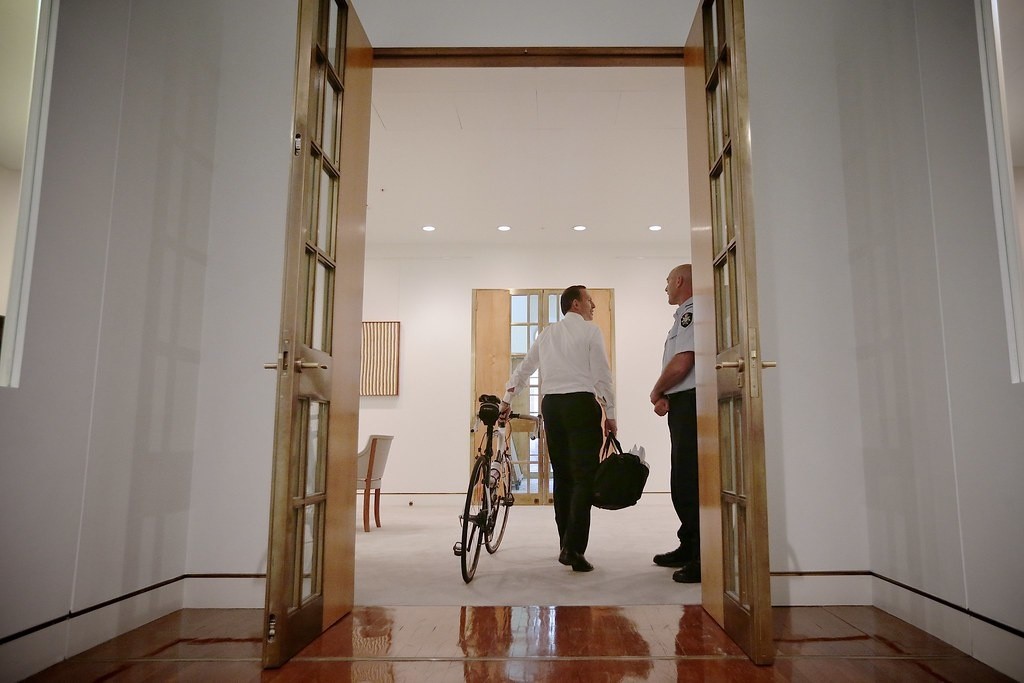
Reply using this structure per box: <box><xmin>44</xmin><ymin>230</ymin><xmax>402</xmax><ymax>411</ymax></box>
<box><xmin>590</xmin><ymin>432</ymin><xmax>650</xmax><ymax>510</ymax></box>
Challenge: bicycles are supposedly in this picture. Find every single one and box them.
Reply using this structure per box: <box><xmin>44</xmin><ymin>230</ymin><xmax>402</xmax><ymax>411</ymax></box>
<box><xmin>453</xmin><ymin>395</ymin><xmax>545</xmax><ymax>586</ymax></box>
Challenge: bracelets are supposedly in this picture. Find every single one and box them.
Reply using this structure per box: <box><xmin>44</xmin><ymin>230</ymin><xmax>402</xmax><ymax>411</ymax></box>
<box><xmin>502</xmin><ymin>401</ymin><xmax>510</xmax><ymax>407</ymax></box>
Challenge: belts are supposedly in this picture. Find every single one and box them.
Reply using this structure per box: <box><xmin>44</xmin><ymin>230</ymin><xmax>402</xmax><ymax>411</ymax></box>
<box><xmin>666</xmin><ymin>393</ymin><xmax>678</xmax><ymax>399</ymax></box>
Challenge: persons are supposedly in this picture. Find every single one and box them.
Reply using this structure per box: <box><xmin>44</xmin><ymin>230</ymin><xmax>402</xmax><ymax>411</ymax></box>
<box><xmin>650</xmin><ymin>264</ymin><xmax>701</xmax><ymax>583</ymax></box>
<box><xmin>500</xmin><ymin>285</ymin><xmax>618</xmax><ymax>572</ymax></box>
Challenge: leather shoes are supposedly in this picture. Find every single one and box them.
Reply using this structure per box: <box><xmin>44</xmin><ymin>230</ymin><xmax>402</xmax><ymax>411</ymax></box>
<box><xmin>673</xmin><ymin>564</ymin><xmax>701</xmax><ymax>583</ymax></box>
<box><xmin>653</xmin><ymin>548</ymin><xmax>688</xmax><ymax>567</ymax></box>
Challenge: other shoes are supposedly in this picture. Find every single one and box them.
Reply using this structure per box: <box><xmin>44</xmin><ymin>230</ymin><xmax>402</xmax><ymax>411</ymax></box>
<box><xmin>559</xmin><ymin>547</ymin><xmax>570</xmax><ymax>565</ymax></box>
<box><xmin>566</xmin><ymin>547</ymin><xmax>594</xmax><ymax>572</ymax></box>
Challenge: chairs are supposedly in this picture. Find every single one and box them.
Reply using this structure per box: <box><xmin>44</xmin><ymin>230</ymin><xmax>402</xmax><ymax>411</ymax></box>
<box><xmin>356</xmin><ymin>435</ymin><xmax>395</xmax><ymax>532</ymax></box>
